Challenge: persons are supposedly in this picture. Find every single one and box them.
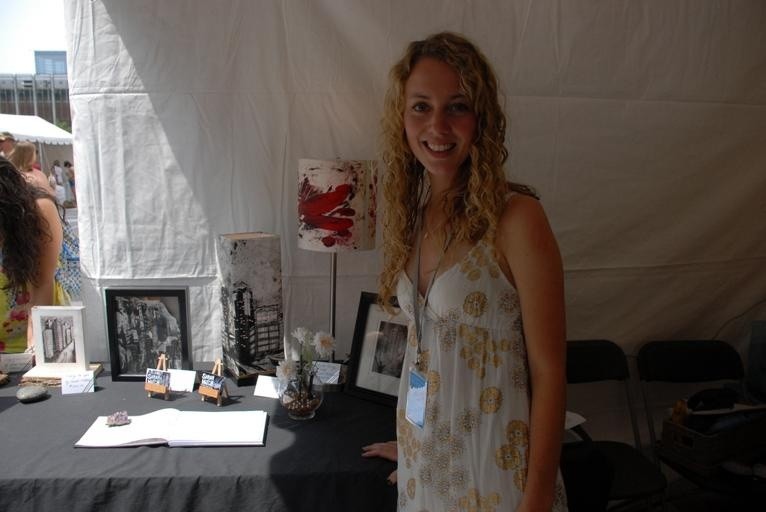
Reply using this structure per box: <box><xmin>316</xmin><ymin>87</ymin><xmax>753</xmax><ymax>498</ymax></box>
<box><xmin>50</xmin><ymin>159</ymin><xmax>66</xmax><ymax>204</ymax></box>
<box><xmin>5</xmin><ymin>141</ymin><xmax>50</xmax><ymax>189</ymax></box>
<box><xmin>0</xmin><ymin>131</ymin><xmax>17</xmax><ymax>157</ymax></box>
<box><xmin>62</xmin><ymin>159</ymin><xmax>76</xmax><ymax>208</ymax></box>
<box><xmin>0</xmin><ymin>154</ymin><xmax>79</xmax><ymax>355</ymax></box>
<box><xmin>360</xmin><ymin>30</ymin><xmax>578</xmax><ymax>512</ymax></box>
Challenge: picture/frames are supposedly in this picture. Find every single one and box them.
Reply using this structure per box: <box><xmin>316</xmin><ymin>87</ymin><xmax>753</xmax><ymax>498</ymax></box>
<box><xmin>105</xmin><ymin>284</ymin><xmax>191</xmax><ymax>383</ymax></box>
<box><xmin>30</xmin><ymin>303</ymin><xmax>91</xmax><ymax>371</ymax></box>
<box><xmin>341</xmin><ymin>288</ymin><xmax>410</xmax><ymax>409</ymax></box>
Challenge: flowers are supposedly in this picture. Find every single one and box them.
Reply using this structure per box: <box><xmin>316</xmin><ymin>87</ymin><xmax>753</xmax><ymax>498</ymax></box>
<box><xmin>273</xmin><ymin>326</ymin><xmax>339</xmax><ymax>401</ymax></box>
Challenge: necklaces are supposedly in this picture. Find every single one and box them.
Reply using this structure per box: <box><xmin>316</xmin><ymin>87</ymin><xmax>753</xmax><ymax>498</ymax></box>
<box><xmin>422</xmin><ymin>216</ymin><xmax>449</xmax><ymax>241</ymax></box>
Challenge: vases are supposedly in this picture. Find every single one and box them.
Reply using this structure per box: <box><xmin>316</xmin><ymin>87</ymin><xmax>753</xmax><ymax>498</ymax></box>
<box><xmin>277</xmin><ymin>364</ymin><xmax>326</xmax><ymax>423</ymax></box>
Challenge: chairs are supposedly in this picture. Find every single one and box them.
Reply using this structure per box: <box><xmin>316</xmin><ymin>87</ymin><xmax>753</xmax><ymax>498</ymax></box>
<box><xmin>635</xmin><ymin>335</ymin><xmax>766</xmax><ymax>510</ymax></box>
<box><xmin>747</xmin><ymin>318</ymin><xmax>766</xmax><ymax>404</ymax></box>
<box><xmin>559</xmin><ymin>337</ymin><xmax>669</xmax><ymax>511</ymax></box>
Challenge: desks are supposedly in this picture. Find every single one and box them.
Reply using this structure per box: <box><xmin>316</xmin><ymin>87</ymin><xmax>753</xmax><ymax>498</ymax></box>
<box><xmin>0</xmin><ymin>369</ymin><xmax>397</xmax><ymax>511</ymax></box>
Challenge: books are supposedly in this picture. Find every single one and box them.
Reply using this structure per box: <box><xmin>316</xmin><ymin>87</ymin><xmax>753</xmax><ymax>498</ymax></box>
<box><xmin>73</xmin><ymin>408</ymin><xmax>268</xmax><ymax>450</ymax></box>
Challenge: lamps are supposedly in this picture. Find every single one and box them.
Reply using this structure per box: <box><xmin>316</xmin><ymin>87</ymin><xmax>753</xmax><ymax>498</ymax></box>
<box><xmin>295</xmin><ymin>154</ymin><xmax>380</xmax><ymax>364</ymax></box>
